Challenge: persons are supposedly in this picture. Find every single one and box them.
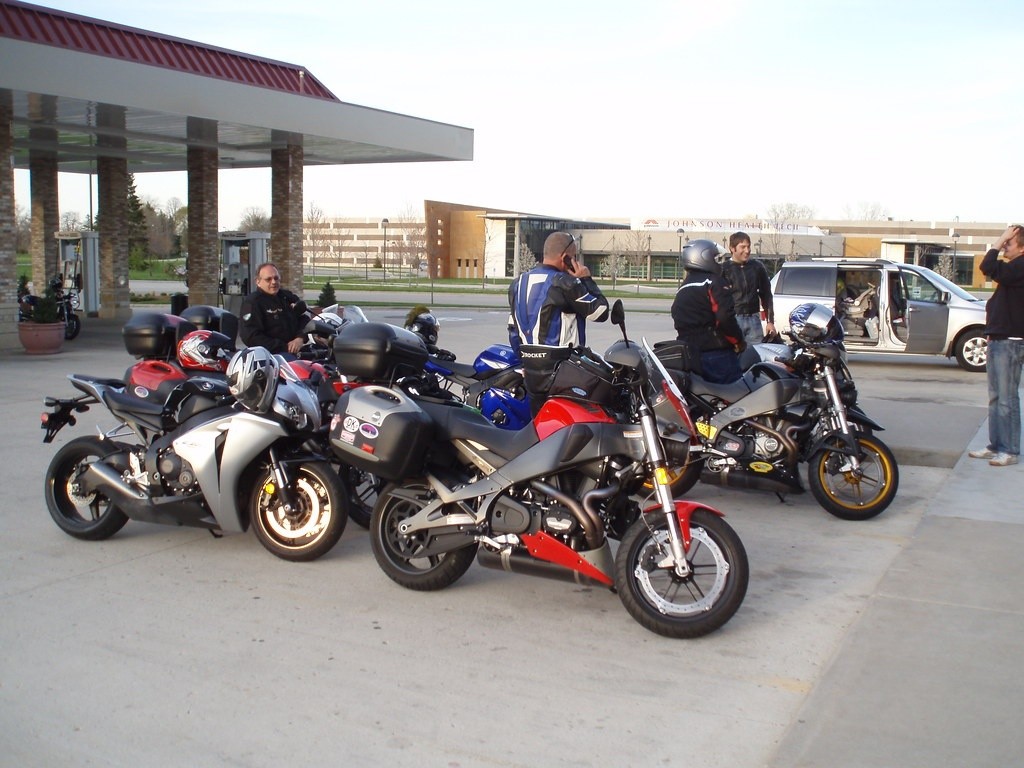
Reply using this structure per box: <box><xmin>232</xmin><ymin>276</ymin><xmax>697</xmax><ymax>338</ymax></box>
<box><xmin>968</xmin><ymin>224</ymin><xmax>1024</xmax><ymax>466</ymax></box>
<box><xmin>671</xmin><ymin>239</ymin><xmax>763</xmax><ymax>384</ymax></box>
<box><xmin>222</xmin><ymin>276</ymin><xmax>249</xmax><ymax>294</ymax></box>
<box><xmin>507</xmin><ymin>231</ymin><xmax>609</xmax><ymax>421</ymax></box>
<box><xmin>239</xmin><ymin>262</ymin><xmax>311</xmax><ymax>362</ymax></box>
<box><xmin>722</xmin><ymin>232</ymin><xmax>776</xmax><ymax>346</ymax></box>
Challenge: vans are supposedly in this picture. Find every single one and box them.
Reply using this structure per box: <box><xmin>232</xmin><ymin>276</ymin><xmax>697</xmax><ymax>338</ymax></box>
<box><xmin>759</xmin><ymin>257</ymin><xmax>989</xmax><ymax>373</ymax></box>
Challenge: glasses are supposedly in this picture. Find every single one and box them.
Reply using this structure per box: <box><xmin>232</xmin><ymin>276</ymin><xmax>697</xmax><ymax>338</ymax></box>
<box><xmin>260</xmin><ymin>275</ymin><xmax>281</xmax><ymax>284</ymax></box>
<box><xmin>560</xmin><ymin>232</ymin><xmax>576</xmax><ymax>257</ymax></box>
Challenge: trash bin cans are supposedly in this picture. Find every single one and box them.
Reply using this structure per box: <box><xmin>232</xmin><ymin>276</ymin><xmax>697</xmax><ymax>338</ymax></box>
<box><xmin>172</xmin><ymin>293</ymin><xmax>188</xmax><ymax>315</ymax></box>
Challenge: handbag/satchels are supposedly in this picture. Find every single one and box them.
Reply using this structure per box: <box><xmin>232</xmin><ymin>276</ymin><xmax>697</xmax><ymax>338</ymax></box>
<box><xmin>865</xmin><ymin>317</ymin><xmax>880</xmax><ymax>340</ymax></box>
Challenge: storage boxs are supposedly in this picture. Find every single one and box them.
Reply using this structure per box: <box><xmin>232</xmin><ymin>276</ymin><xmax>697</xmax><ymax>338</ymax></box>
<box><xmin>327</xmin><ymin>384</ymin><xmax>433</xmax><ymax>480</ymax></box>
<box><xmin>179</xmin><ymin>303</ymin><xmax>239</xmax><ymax>342</ymax></box>
<box><xmin>652</xmin><ymin>339</ymin><xmax>691</xmax><ymax>370</ymax></box>
<box><xmin>330</xmin><ymin>321</ymin><xmax>428</xmax><ymax>379</ymax></box>
<box><xmin>119</xmin><ymin>312</ymin><xmax>198</xmax><ymax>357</ymax></box>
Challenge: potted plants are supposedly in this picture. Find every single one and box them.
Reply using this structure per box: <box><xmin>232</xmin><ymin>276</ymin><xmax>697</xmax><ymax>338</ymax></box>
<box><xmin>18</xmin><ymin>285</ymin><xmax>67</xmax><ymax>354</ymax></box>
<box><xmin>310</xmin><ymin>282</ymin><xmax>344</xmax><ymax>324</ymax></box>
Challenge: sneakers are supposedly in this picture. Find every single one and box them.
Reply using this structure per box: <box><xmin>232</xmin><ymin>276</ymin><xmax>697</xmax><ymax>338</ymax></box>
<box><xmin>969</xmin><ymin>447</ymin><xmax>997</xmax><ymax>459</ymax></box>
<box><xmin>988</xmin><ymin>451</ymin><xmax>1019</xmax><ymax>465</ymax></box>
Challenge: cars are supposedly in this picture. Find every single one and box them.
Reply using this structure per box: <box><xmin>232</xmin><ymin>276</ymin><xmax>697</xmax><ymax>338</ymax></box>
<box><xmin>419</xmin><ymin>262</ymin><xmax>427</xmax><ymax>271</ymax></box>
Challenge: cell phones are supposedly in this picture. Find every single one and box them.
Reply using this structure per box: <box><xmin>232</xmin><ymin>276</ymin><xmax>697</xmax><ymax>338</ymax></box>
<box><xmin>563</xmin><ymin>255</ymin><xmax>576</xmax><ymax>274</ymax></box>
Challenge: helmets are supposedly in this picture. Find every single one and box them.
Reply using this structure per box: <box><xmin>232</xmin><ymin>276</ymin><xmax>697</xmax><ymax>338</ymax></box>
<box><xmin>682</xmin><ymin>240</ymin><xmax>723</xmax><ymax>276</ymax></box>
<box><xmin>225</xmin><ymin>346</ymin><xmax>280</xmax><ymax>414</ymax></box>
<box><xmin>177</xmin><ymin>329</ymin><xmax>236</xmax><ymax>373</ymax></box>
<box><xmin>48</xmin><ymin>278</ymin><xmax>63</xmax><ymax>290</ymax></box>
<box><xmin>404</xmin><ymin>305</ymin><xmax>439</xmax><ymax>345</ymax></box>
<box><xmin>790</xmin><ymin>303</ymin><xmax>844</xmax><ymax>347</ymax></box>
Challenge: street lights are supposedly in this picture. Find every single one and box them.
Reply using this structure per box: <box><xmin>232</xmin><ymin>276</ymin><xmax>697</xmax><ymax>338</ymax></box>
<box><xmin>677</xmin><ymin>228</ymin><xmax>684</xmax><ymax>289</ymax></box>
<box><xmin>387</xmin><ymin>238</ymin><xmax>395</xmax><ymax>276</ymax></box>
<box><xmin>382</xmin><ymin>217</ymin><xmax>389</xmax><ymax>282</ymax></box>
<box><xmin>951</xmin><ymin>232</ymin><xmax>960</xmax><ymax>283</ymax></box>
<box><xmin>754</xmin><ymin>243</ymin><xmax>760</xmax><ymax>259</ymax></box>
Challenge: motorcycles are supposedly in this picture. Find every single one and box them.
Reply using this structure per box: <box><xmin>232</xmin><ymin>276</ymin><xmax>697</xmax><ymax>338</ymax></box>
<box><xmin>121</xmin><ymin>278</ymin><xmax>899</xmax><ymax>520</ymax></box>
<box><xmin>329</xmin><ymin>298</ymin><xmax>750</xmax><ymax>642</ymax></box>
<box><xmin>40</xmin><ymin>355</ymin><xmax>348</xmax><ymax>563</ymax></box>
<box><xmin>19</xmin><ymin>279</ymin><xmax>81</xmax><ymax>339</ymax></box>
